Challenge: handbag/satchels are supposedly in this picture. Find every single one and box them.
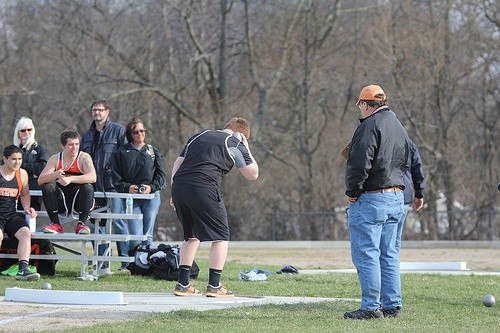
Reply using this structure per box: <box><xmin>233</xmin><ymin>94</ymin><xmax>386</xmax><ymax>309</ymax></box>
<box><xmin>0</xmin><ymin>238</ymin><xmax>58</xmax><ymax>276</ymax></box>
<box><xmin>128</xmin><ymin>241</ymin><xmax>200</xmax><ymax>280</ymax></box>
<box><xmin>148</xmin><ymin>144</ymin><xmax>168</xmax><ymax>190</ymax></box>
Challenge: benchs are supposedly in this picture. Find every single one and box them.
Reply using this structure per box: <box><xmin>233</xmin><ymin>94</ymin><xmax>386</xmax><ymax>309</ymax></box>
<box><xmin>0</xmin><ymin>190</ymin><xmax>156</xmax><ymax>281</ymax></box>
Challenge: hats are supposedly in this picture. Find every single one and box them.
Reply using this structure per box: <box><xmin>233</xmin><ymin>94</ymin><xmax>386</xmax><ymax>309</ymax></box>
<box><xmin>356</xmin><ymin>84</ymin><xmax>386</xmax><ymax>105</ymax></box>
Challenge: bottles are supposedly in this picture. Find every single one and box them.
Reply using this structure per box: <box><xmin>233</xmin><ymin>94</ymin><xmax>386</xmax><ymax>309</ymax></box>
<box><xmin>125</xmin><ymin>194</ymin><xmax>133</xmax><ymax>216</ymax></box>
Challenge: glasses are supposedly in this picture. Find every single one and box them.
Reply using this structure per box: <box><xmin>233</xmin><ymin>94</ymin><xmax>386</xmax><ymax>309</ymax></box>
<box><xmin>132</xmin><ymin>129</ymin><xmax>145</xmax><ymax>135</ymax></box>
<box><xmin>20</xmin><ymin>129</ymin><xmax>33</xmax><ymax>132</ymax></box>
<box><xmin>92</xmin><ymin>108</ymin><xmax>105</xmax><ymax>112</ymax></box>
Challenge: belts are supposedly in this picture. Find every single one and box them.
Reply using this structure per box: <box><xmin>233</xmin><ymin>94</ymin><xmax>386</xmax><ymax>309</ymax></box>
<box><xmin>370</xmin><ymin>187</ymin><xmax>401</xmax><ymax>192</ymax></box>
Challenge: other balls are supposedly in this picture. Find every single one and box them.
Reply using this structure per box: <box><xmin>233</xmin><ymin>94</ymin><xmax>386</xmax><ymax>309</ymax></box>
<box><xmin>233</xmin><ymin>132</ymin><xmax>242</xmax><ymax>140</ymax></box>
<box><xmin>43</xmin><ymin>283</ymin><xmax>51</xmax><ymax>289</ymax></box>
<box><xmin>483</xmin><ymin>295</ymin><xmax>495</xmax><ymax>307</ymax></box>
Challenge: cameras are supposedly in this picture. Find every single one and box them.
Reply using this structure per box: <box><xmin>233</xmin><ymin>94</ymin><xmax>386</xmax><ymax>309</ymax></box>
<box><xmin>135</xmin><ymin>184</ymin><xmax>146</xmax><ymax>194</ymax></box>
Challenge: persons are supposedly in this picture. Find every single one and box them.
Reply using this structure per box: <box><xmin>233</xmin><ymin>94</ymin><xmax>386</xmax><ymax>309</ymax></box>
<box><xmin>114</xmin><ymin>118</ymin><xmax>166</xmax><ymax>270</ymax></box>
<box><xmin>169</xmin><ymin>115</ymin><xmax>260</xmax><ymax>298</ymax></box>
<box><xmin>396</xmin><ymin>136</ymin><xmax>426</xmax><ymax>252</ymax></box>
<box><xmin>80</xmin><ymin>99</ymin><xmax>128</xmax><ymax>275</ymax></box>
<box><xmin>0</xmin><ymin>145</ymin><xmax>41</xmax><ymax>281</ymax></box>
<box><xmin>37</xmin><ymin>129</ymin><xmax>97</xmax><ymax>234</ymax></box>
<box><xmin>13</xmin><ymin>116</ymin><xmax>48</xmax><ymax>211</ymax></box>
<box><xmin>343</xmin><ymin>84</ymin><xmax>412</xmax><ymax>320</ymax></box>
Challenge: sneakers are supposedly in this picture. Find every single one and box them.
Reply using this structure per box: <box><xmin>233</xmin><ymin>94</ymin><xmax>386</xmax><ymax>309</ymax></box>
<box><xmin>383</xmin><ymin>306</ymin><xmax>401</xmax><ymax>317</ymax></box>
<box><xmin>344</xmin><ymin>309</ymin><xmax>383</xmax><ymax>319</ymax></box>
<box><xmin>174</xmin><ymin>281</ymin><xmax>200</xmax><ymax>295</ymax></box>
<box><xmin>15</xmin><ymin>268</ymin><xmax>40</xmax><ymax>281</ymax></box>
<box><xmin>43</xmin><ymin>223</ymin><xmax>64</xmax><ymax>233</ymax></box>
<box><xmin>75</xmin><ymin>224</ymin><xmax>90</xmax><ymax>234</ymax></box>
<box><xmin>206</xmin><ymin>283</ymin><xmax>233</xmax><ymax>297</ymax></box>
